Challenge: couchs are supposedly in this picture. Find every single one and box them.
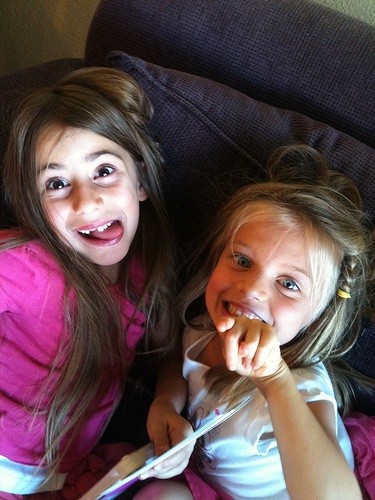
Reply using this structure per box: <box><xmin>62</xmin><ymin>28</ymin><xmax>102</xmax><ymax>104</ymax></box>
<box><xmin>0</xmin><ymin>0</ymin><xmax>375</xmax><ymax>448</ymax></box>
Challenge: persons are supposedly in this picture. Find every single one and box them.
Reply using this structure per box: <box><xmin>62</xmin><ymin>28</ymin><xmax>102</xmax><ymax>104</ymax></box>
<box><xmin>131</xmin><ymin>143</ymin><xmax>375</xmax><ymax>500</ymax></box>
<box><xmin>0</xmin><ymin>66</ymin><xmax>196</xmax><ymax>500</ymax></box>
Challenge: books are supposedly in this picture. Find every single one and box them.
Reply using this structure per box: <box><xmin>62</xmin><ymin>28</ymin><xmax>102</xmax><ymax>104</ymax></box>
<box><xmin>79</xmin><ymin>394</ymin><xmax>256</xmax><ymax>500</ymax></box>
